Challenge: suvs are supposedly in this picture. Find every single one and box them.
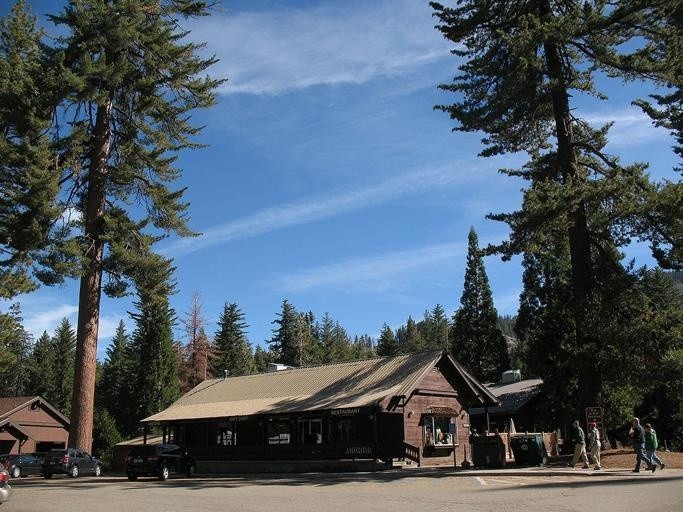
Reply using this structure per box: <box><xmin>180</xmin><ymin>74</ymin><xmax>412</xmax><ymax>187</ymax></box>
<box><xmin>39</xmin><ymin>447</ymin><xmax>104</xmax><ymax>480</ymax></box>
<box><xmin>126</xmin><ymin>444</ymin><xmax>195</xmax><ymax>481</ymax></box>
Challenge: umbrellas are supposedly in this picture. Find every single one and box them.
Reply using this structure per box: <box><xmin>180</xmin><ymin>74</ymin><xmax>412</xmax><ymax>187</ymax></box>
<box><xmin>508</xmin><ymin>416</ymin><xmax>516</xmax><ymax>461</ymax></box>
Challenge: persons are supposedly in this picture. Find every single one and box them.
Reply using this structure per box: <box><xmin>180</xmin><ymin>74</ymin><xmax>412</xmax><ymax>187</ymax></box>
<box><xmin>435</xmin><ymin>426</ymin><xmax>441</xmax><ymax>443</ymax></box>
<box><xmin>425</xmin><ymin>427</ymin><xmax>432</xmax><ymax>443</ymax></box>
<box><xmin>602</xmin><ymin>435</ymin><xmax>611</xmax><ymax>450</ymax></box>
<box><xmin>644</xmin><ymin>423</ymin><xmax>665</xmax><ymax>470</ymax></box>
<box><xmin>567</xmin><ymin>420</ymin><xmax>589</xmax><ymax>468</ymax></box>
<box><xmin>632</xmin><ymin>417</ymin><xmax>657</xmax><ymax>472</ymax></box>
<box><xmin>588</xmin><ymin>422</ymin><xmax>601</xmax><ymax>470</ymax></box>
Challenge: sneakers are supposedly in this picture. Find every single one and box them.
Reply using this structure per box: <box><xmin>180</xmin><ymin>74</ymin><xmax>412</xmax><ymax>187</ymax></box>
<box><xmin>567</xmin><ymin>463</ymin><xmax>600</xmax><ymax>469</ymax></box>
<box><xmin>632</xmin><ymin>464</ymin><xmax>664</xmax><ymax>472</ymax></box>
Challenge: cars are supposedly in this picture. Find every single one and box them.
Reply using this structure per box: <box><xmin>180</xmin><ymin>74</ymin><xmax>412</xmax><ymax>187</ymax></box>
<box><xmin>0</xmin><ymin>462</ymin><xmax>12</xmax><ymax>506</ymax></box>
<box><xmin>0</xmin><ymin>453</ymin><xmax>44</xmax><ymax>479</ymax></box>
